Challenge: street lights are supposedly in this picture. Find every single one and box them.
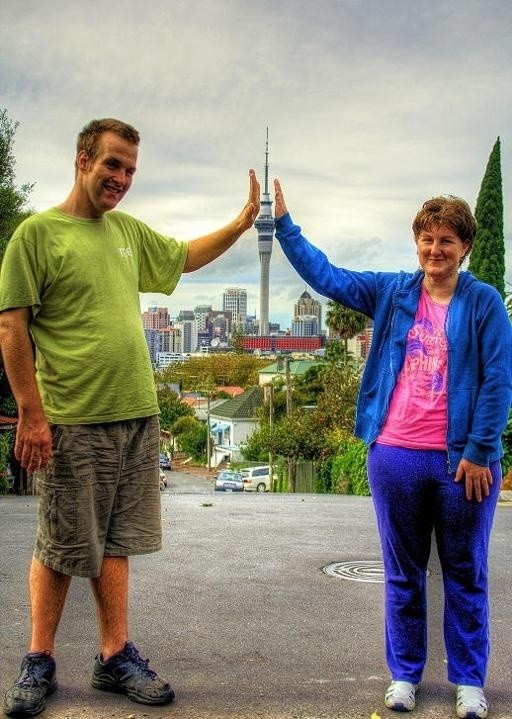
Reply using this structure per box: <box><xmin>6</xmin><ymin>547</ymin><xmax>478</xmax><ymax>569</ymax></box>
<box><xmin>262</xmin><ymin>383</ymin><xmax>273</xmax><ymax>491</ymax></box>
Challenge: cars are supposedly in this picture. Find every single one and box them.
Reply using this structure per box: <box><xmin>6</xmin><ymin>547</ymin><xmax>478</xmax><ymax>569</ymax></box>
<box><xmin>159</xmin><ymin>451</ymin><xmax>171</xmax><ymax>471</ymax></box>
<box><xmin>213</xmin><ymin>471</ymin><xmax>244</xmax><ymax>493</ymax></box>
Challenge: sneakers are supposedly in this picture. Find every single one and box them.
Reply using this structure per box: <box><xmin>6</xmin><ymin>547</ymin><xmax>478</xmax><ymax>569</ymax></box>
<box><xmin>384</xmin><ymin>680</ymin><xmax>419</xmax><ymax>712</ymax></box>
<box><xmin>455</xmin><ymin>684</ymin><xmax>488</xmax><ymax>719</ymax></box>
<box><xmin>4</xmin><ymin>652</ymin><xmax>59</xmax><ymax>718</ymax></box>
<box><xmin>91</xmin><ymin>640</ymin><xmax>175</xmax><ymax>706</ymax></box>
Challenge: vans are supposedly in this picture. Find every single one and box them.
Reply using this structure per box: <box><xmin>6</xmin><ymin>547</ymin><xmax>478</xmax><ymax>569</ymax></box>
<box><xmin>241</xmin><ymin>465</ymin><xmax>280</xmax><ymax>492</ymax></box>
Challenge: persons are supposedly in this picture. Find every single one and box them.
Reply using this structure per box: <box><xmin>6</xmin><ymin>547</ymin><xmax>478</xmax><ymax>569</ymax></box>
<box><xmin>0</xmin><ymin>118</ymin><xmax>261</xmax><ymax>718</ymax></box>
<box><xmin>274</xmin><ymin>178</ymin><xmax>512</xmax><ymax>718</ymax></box>
